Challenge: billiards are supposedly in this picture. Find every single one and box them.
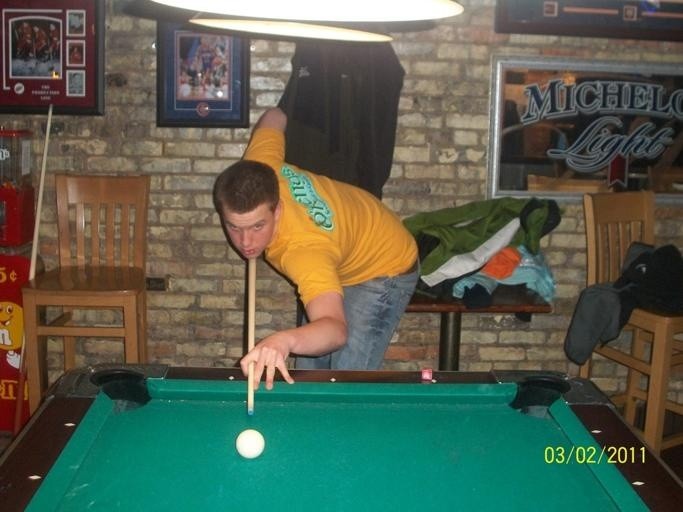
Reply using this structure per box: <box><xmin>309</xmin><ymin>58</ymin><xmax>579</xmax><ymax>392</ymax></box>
<box><xmin>236</xmin><ymin>429</ymin><xmax>265</xmax><ymax>458</ymax></box>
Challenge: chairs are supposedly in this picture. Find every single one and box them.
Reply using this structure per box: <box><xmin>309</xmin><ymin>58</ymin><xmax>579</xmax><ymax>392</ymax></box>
<box><xmin>21</xmin><ymin>174</ymin><xmax>151</xmax><ymax>417</ymax></box>
<box><xmin>576</xmin><ymin>188</ymin><xmax>683</xmax><ymax>462</ymax></box>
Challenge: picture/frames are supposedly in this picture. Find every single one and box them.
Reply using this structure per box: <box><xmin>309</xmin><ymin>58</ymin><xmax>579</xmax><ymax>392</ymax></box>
<box><xmin>0</xmin><ymin>1</ymin><xmax>104</xmax><ymax>117</ymax></box>
<box><xmin>486</xmin><ymin>5</ymin><xmax>682</xmax><ymax>208</ymax></box>
<box><xmin>154</xmin><ymin>19</ymin><xmax>249</xmax><ymax>128</ymax></box>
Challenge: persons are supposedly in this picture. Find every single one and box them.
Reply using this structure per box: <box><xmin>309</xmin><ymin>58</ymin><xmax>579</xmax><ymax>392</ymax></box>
<box><xmin>13</xmin><ymin>21</ymin><xmax>33</xmax><ymax>62</ymax></box>
<box><xmin>43</xmin><ymin>23</ymin><xmax>60</xmax><ymax>60</ymax></box>
<box><xmin>68</xmin><ymin>72</ymin><xmax>83</xmax><ymax>95</ymax></box>
<box><xmin>70</xmin><ymin>47</ymin><xmax>82</xmax><ymax>64</ymax></box>
<box><xmin>26</xmin><ymin>25</ymin><xmax>48</xmax><ymax>59</ymax></box>
<box><xmin>211</xmin><ymin>104</ymin><xmax>420</xmax><ymax>391</ymax></box>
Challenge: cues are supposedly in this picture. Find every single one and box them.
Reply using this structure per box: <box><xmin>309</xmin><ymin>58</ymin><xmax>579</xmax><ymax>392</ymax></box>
<box><xmin>248</xmin><ymin>258</ymin><xmax>256</xmax><ymax>416</ymax></box>
<box><xmin>564</xmin><ymin>8</ymin><xmax>681</xmax><ymax>18</ymax></box>
<box><xmin>13</xmin><ymin>103</ymin><xmax>52</xmax><ymax>441</ymax></box>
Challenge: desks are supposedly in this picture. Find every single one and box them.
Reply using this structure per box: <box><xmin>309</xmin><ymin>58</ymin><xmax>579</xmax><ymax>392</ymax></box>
<box><xmin>0</xmin><ymin>367</ymin><xmax>679</xmax><ymax>512</ymax></box>
<box><xmin>402</xmin><ymin>284</ymin><xmax>552</xmax><ymax>371</ymax></box>
